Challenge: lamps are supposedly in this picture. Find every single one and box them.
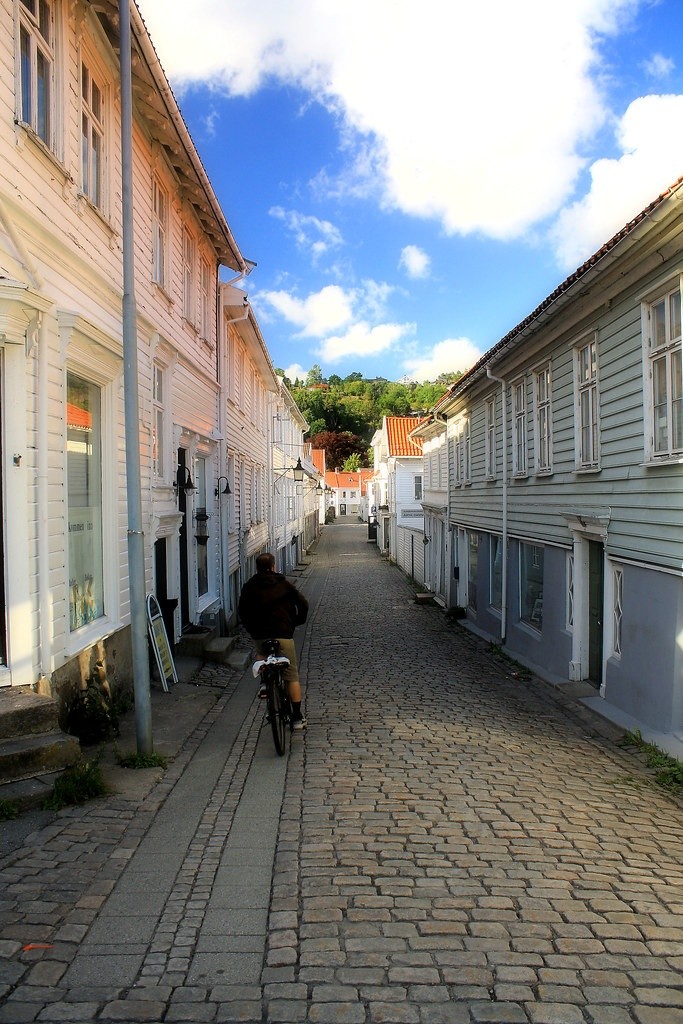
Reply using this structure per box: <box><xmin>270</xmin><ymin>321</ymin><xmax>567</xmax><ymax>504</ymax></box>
<box><xmin>423</xmin><ymin>534</ymin><xmax>432</xmax><ymax>545</ymax></box>
<box><xmin>273</xmin><ymin>457</ymin><xmax>306</xmax><ymax>495</ymax></box>
<box><xmin>192</xmin><ymin>508</ymin><xmax>215</xmax><ymax>545</ymax></box>
<box><xmin>215</xmin><ymin>476</ymin><xmax>232</xmax><ymax>500</ymax></box>
<box><xmin>173</xmin><ymin>466</ymin><xmax>198</xmax><ymax>496</ymax></box>
<box><xmin>303</xmin><ymin>481</ymin><xmax>322</xmax><ymax>498</ymax></box>
<box><xmin>323</xmin><ymin>485</ymin><xmax>334</xmax><ymax>494</ymax></box>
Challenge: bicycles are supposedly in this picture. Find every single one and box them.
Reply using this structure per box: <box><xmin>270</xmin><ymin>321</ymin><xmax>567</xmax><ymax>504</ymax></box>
<box><xmin>255</xmin><ymin>638</ymin><xmax>292</xmax><ymax>757</ymax></box>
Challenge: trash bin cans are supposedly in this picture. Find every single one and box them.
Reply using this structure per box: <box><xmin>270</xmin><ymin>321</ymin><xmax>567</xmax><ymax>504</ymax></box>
<box><xmin>200</xmin><ymin>604</ymin><xmax>223</xmax><ymax>637</ymax></box>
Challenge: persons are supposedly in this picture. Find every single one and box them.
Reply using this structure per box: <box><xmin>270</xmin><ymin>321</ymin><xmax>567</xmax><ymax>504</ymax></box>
<box><xmin>238</xmin><ymin>553</ymin><xmax>308</xmax><ymax>729</ymax></box>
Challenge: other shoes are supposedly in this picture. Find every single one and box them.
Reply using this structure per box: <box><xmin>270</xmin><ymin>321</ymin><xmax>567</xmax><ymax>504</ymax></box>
<box><xmin>293</xmin><ymin>718</ymin><xmax>308</xmax><ymax>730</ymax></box>
<box><xmin>257</xmin><ymin>685</ymin><xmax>266</xmax><ymax>698</ymax></box>
